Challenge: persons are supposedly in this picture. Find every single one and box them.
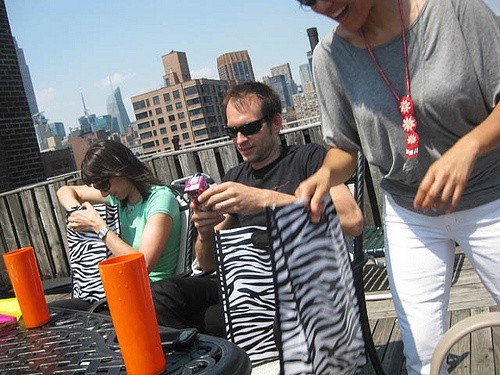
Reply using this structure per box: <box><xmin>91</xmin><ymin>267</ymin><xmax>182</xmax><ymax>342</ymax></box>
<box><xmin>148</xmin><ymin>80</ymin><xmax>364</xmax><ymax>340</ymax></box>
<box><xmin>294</xmin><ymin>0</ymin><xmax>500</xmax><ymax>375</ymax></box>
<box><xmin>46</xmin><ymin>139</ymin><xmax>180</xmax><ymax>315</ymax></box>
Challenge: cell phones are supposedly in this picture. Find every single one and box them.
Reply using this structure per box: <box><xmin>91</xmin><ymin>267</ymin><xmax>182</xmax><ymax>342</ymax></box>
<box><xmin>107</xmin><ymin>328</ymin><xmax>198</xmax><ymax>349</ymax></box>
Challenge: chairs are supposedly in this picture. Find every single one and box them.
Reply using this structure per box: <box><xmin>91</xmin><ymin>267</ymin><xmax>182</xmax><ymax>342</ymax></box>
<box><xmin>175</xmin><ymin>173</ymin><xmax>218</xmax><ymax>277</ymax></box>
<box><xmin>339</xmin><ymin>151</ymin><xmax>385</xmax><ymax>375</ymax></box>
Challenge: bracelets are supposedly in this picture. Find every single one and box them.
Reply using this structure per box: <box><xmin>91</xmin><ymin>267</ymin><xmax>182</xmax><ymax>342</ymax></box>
<box><xmin>66</xmin><ymin>206</ymin><xmax>81</xmax><ymax>225</ymax></box>
<box><xmin>97</xmin><ymin>227</ymin><xmax>109</xmax><ymax>240</ymax></box>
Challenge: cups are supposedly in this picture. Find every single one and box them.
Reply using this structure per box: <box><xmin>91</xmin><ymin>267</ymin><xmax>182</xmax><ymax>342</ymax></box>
<box><xmin>98</xmin><ymin>253</ymin><xmax>166</xmax><ymax>375</ymax></box>
<box><xmin>3</xmin><ymin>247</ymin><xmax>50</xmax><ymax>329</ymax></box>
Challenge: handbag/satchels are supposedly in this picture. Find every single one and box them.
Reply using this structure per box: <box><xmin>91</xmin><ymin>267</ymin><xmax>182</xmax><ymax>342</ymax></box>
<box><xmin>213</xmin><ymin>196</ymin><xmax>367</xmax><ymax>375</ymax></box>
<box><xmin>64</xmin><ymin>203</ymin><xmax>119</xmax><ymax>302</ymax></box>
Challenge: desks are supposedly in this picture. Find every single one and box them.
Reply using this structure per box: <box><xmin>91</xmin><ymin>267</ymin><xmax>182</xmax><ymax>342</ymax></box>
<box><xmin>0</xmin><ymin>301</ymin><xmax>253</xmax><ymax>375</ymax></box>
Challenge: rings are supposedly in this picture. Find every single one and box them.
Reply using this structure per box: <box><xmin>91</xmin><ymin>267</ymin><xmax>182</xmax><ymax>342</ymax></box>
<box><xmin>221</xmin><ymin>202</ymin><xmax>223</xmax><ymax>210</ymax></box>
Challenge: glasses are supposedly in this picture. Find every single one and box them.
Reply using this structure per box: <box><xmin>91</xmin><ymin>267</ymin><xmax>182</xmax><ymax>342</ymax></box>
<box><xmin>93</xmin><ymin>174</ymin><xmax>113</xmax><ymax>193</ymax></box>
<box><xmin>224</xmin><ymin>112</ymin><xmax>275</xmax><ymax>138</ymax></box>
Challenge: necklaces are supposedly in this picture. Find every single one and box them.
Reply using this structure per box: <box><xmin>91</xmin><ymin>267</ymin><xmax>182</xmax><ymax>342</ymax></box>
<box><xmin>359</xmin><ymin>1</ymin><xmax>418</xmax><ymax>158</ymax></box>
